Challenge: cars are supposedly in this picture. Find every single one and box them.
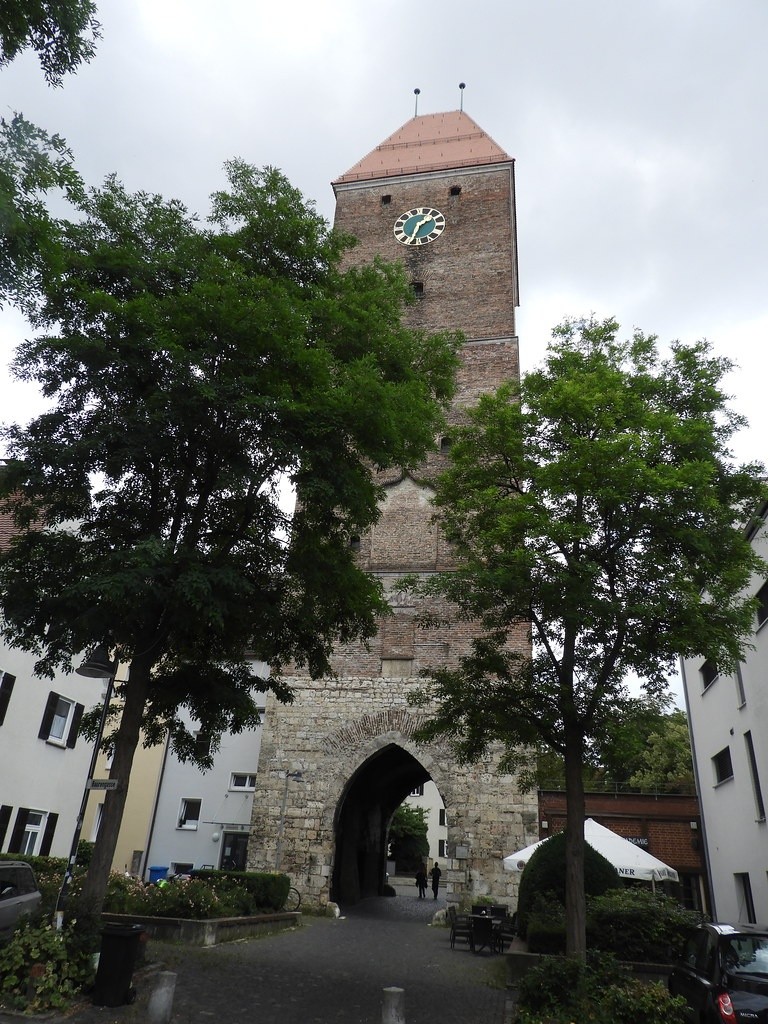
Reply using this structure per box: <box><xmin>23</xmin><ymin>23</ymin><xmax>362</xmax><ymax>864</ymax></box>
<box><xmin>667</xmin><ymin>921</ymin><xmax>768</xmax><ymax>1024</ymax></box>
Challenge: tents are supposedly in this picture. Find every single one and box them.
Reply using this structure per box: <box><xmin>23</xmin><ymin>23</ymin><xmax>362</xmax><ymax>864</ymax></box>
<box><xmin>503</xmin><ymin>818</ymin><xmax>679</xmax><ymax>897</ymax></box>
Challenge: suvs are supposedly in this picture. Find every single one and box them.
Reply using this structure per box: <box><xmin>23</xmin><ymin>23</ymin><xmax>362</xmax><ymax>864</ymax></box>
<box><xmin>0</xmin><ymin>861</ymin><xmax>44</xmax><ymax>951</ymax></box>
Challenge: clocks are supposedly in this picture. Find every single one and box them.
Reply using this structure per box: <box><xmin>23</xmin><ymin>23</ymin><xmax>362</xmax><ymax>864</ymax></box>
<box><xmin>394</xmin><ymin>207</ymin><xmax>445</xmax><ymax>246</ymax></box>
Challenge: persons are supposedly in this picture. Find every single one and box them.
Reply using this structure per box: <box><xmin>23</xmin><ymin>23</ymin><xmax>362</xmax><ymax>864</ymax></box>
<box><xmin>428</xmin><ymin>862</ymin><xmax>442</xmax><ymax>900</ymax></box>
<box><xmin>414</xmin><ymin>863</ymin><xmax>428</xmax><ymax>899</ymax></box>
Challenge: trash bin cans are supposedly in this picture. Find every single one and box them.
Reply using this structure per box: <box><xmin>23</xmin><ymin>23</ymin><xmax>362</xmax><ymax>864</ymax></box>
<box><xmin>148</xmin><ymin>866</ymin><xmax>169</xmax><ymax>884</ymax></box>
<box><xmin>92</xmin><ymin>921</ymin><xmax>145</xmax><ymax>1007</ymax></box>
<box><xmin>174</xmin><ymin>862</ymin><xmax>193</xmax><ymax>875</ymax></box>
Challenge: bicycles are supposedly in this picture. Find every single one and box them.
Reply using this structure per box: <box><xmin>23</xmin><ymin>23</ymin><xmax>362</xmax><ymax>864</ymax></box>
<box><xmin>199</xmin><ymin>859</ymin><xmax>301</xmax><ymax>913</ymax></box>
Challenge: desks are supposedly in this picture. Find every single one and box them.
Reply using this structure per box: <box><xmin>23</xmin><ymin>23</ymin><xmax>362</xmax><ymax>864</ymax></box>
<box><xmin>469</xmin><ymin>914</ymin><xmax>495</xmax><ymax>920</ymax></box>
<box><xmin>476</xmin><ymin>920</ymin><xmax>502</xmax><ymax>955</ymax></box>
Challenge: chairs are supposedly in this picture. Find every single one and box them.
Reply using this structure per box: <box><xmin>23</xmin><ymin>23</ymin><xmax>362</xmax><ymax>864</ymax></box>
<box><xmin>446</xmin><ymin>903</ymin><xmax>518</xmax><ymax>956</ymax></box>
